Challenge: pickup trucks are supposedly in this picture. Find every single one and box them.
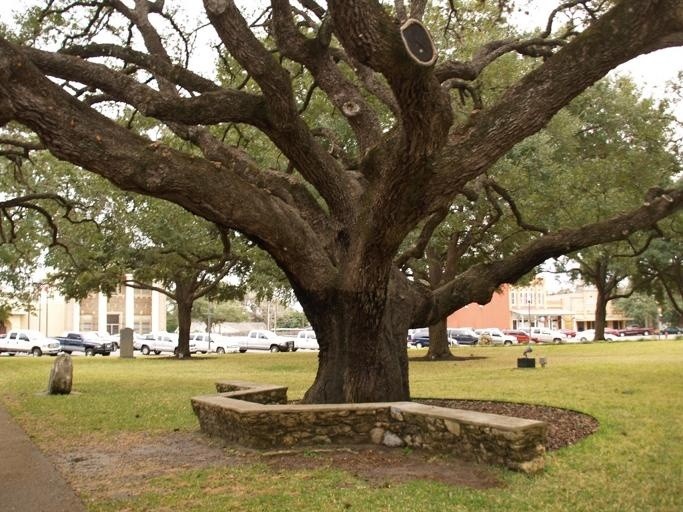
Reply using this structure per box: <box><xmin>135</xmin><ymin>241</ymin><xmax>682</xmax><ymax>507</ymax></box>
<box><xmin>52</xmin><ymin>333</ymin><xmax>114</xmax><ymax>356</ymax></box>
<box><xmin>104</xmin><ymin>330</ymin><xmax>295</xmax><ymax>354</ymax></box>
<box><xmin>0</xmin><ymin>328</ymin><xmax>60</xmax><ymax>357</ymax></box>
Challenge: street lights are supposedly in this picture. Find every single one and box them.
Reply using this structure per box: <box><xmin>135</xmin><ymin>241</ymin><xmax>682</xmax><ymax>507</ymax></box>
<box><xmin>528</xmin><ymin>298</ymin><xmax>531</xmax><ymax>342</ymax></box>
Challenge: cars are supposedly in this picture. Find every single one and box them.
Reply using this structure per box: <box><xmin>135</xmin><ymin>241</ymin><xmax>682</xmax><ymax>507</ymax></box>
<box><xmin>295</xmin><ymin>327</ymin><xmax>319</xmax><ymax>349</ymax></box>
<box><xmin>407</xmin><ymin>325</ymin><xmax>682</xmax><ymax>350</ymax></box>
